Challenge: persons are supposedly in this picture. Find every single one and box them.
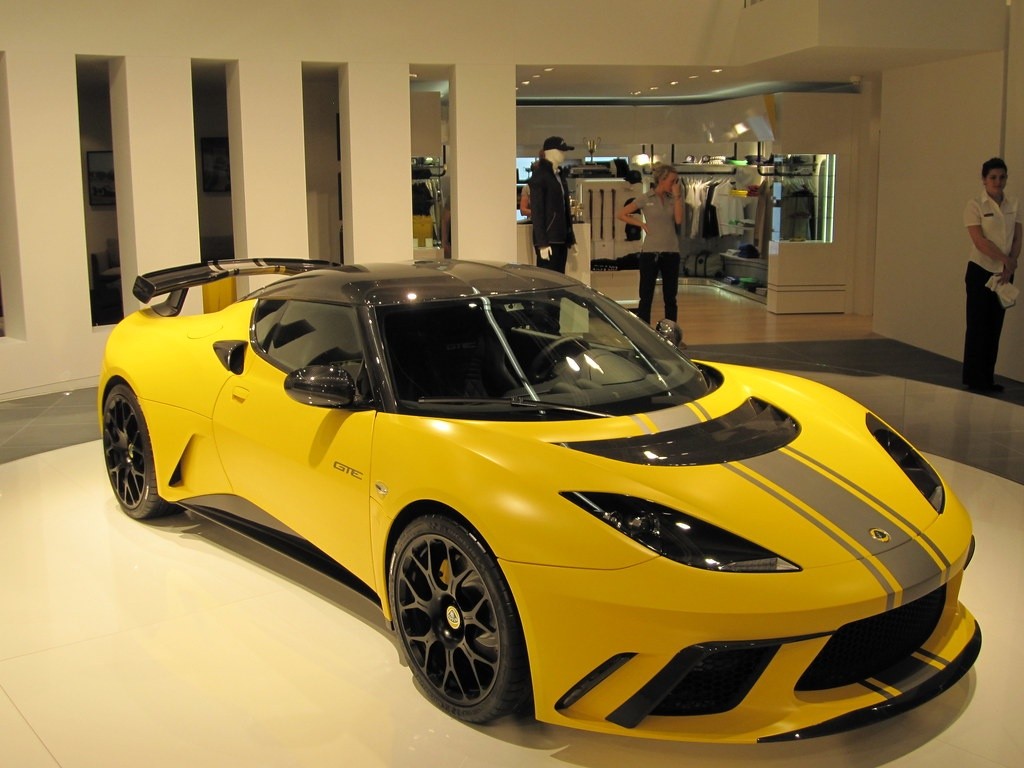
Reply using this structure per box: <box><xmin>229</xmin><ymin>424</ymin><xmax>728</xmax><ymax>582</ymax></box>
<box><xmin>616</xmin><ymin>165</ymin><xmax>688</xmax><ymax>350</ymax></box>
<box><xmin>530</xmin><ymin>136</ymin><xmax>577</xmax><ymax>274</ymax></box>
<box><xmin>520</xmin><ymin>161</ymin><xmax>539</xmax><ymax>220</ymax></box>
<box><xmin>961</xmin><ymin>157</ymin><xmax>1022</xmax><ymax>393</ymax></box>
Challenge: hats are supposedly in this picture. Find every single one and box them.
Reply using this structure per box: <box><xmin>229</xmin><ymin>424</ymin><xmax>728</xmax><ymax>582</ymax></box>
<box><xmin>544</xmin><ymin>136</ymin><xmax>574</xmax><ymax>151</ymax></box>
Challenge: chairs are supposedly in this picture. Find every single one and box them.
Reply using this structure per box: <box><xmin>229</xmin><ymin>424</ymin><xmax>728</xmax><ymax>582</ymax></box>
<box><xmin>483</xmin><ymin>319</ymin><xmax>545</xmax><ymax>400</ymax></box>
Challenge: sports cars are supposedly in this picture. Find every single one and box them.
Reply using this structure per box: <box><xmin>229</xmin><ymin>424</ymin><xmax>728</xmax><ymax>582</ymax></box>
<box><xmin>93</xmin><ymin>250</ymin><xmax>976</xmax><ymax>747</ymax></box>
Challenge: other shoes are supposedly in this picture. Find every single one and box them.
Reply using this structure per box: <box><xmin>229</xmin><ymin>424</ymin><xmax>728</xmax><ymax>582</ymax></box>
<box><xmin>678</xmin><ymin>341</ymin><xmax>687</xmax><ymax>350</ymax></box>
<box><xmin>988</xmin><ymin>382</ymin><xmax>1005</xmax><ymax>392</ymax></box>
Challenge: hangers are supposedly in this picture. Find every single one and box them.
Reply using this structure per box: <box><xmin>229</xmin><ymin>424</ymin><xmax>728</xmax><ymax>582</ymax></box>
<box><xmin>676</xmin><ymin>172</ymin><xmax>817</xmax><ymax>193</ymax></box>
<box><xmin>412</xmin><ymin>178</ymin><xmax>431</xmax><ymax>189</ymax></box>
